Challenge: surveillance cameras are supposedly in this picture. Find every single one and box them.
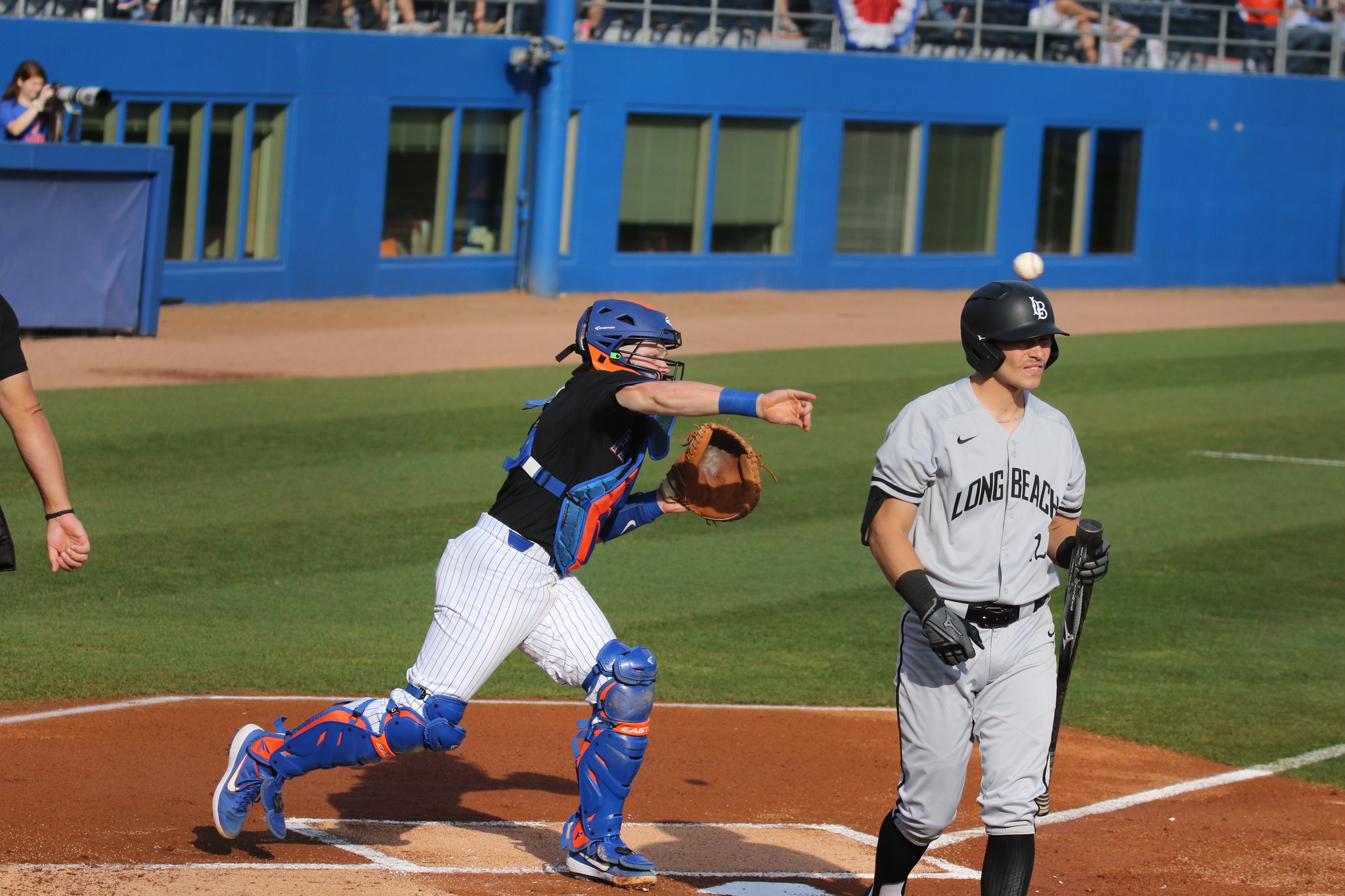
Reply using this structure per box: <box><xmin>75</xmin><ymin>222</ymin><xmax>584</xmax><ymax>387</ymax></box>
<box><xmin>509</xmin><ymin>32</ymin><xmax>565</xmax><ymax>71</ymax></box>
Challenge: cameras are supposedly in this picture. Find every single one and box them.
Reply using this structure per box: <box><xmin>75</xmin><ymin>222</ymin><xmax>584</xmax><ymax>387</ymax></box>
<box><xmin>40</xmin><ymin>82</ymin><xmax>111</xmax><ymax>121</ymax></box>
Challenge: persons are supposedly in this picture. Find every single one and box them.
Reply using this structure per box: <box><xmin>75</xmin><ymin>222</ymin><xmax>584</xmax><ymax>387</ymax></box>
<box><xmin>213</xmin><ymin>296</ymin><xmax>816</xmax><ymax>892</ymax></box>
<box><xmin>467</xmin><ymin>0</ymin><xmax>505</xmax><ymax>35</ymax></box>
<box><xmin>326</xmin><ymin>0</ymin><xmax>440</xmax><ymax>33</ymax></box>
<box><xmin>117</xmin><ymin>0</ymin><xmax>160</xmax><ymax>20</ymax></box>
<box><xmin>1028</xmin><ymin>0</ymin><xmax>1140</xmax><ymax>63</ymax></box>
<box><xmin>0</xmin><ymin>295</ymin><xmax>90</xmax><ymax>573</ymax></box>
<box><xmin>0</xmin><ymin>60</ymin><xmax>62</xmax><ymax>143</ymax></box>
<box><xmin>861</xmin><ymin>280</ymin><xmax>1111</xmax><ymax>896</ymax></box>
<box><xmin>588</xmin><ymin>0</ymin><xmax>606</xmax><ymax>37</ymax></box>
<box><xmin>1236</xmin><ymin>0</ymin><xmax>1345</xmax><ymax>74</ymax></box>
<box><xmin>779</xmin><ymin>0</ymin><xmax>800</xmax><ymax>32</ymax></box>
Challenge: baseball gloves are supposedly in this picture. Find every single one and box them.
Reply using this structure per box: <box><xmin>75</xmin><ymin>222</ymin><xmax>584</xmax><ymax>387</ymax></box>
<box><xmin>660</xmin><ymin>418</ymin><xmax>762</xmax><ymax>523</ymax></box>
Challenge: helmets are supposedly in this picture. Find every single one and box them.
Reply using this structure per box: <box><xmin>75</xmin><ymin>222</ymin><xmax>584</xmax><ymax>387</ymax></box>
<box><xmin>960</xmin><ymin>281</ymin><xmax>1070</xmax><ymax>375</ymax></box>
<box><xmin>577</xmin><ymin>299</ymin><xmax>684</xmax><ymax>381</ymax></box>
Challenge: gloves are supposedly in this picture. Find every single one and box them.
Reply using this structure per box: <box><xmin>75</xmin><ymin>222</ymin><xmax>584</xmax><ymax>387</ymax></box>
<box><xmin>894</xmin><ymin>569</ymin><xmax>985</xmax><ymax>666</ymax></box>
<box><xmin>1056</xmin><ymin>536</ymin><xmax>1110</xmax><ymax>583</ymax></box>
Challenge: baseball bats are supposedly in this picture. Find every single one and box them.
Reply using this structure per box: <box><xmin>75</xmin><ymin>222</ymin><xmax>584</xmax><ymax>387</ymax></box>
<box><xmin>1033</xmin><ymin>519</ymin><xmax>1104</xmax><ymax>817</ymax></box>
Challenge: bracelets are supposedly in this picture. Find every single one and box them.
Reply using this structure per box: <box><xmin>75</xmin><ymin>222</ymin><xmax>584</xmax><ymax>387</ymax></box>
<box><xmin>718</xmin><ymin>387</ymin><xmax>762</xmax><ymax>418</ymax></box>
<box><xmin>32</xmin><ymin>100</ymin><xmax>45</xmax><ymax>111</ymax></box>
<box><xmin>45</xmin><ymin>509</ymin><xmax>74</xmax><ymax>521</ymax></box>
<box><xmin>55</xmin><ymin>116</ymin><xmax>61</xmax><ymax>121</ymax></box>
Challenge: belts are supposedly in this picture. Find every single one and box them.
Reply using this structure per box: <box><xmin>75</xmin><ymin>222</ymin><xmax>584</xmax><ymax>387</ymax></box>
<box><xmin>942</xmin><ymin>593</ymin><xmax>1050</xmax><ymax>629</ymax></box>
<box><xmin>507</xmin><ymin>530</ymin><xmax>554</xmax><ymax>567</ymax></box>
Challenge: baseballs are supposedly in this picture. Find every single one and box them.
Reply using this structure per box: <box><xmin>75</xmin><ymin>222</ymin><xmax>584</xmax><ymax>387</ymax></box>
<box><xmin>1012</xmin><ymin>252</ymin><xmax>1044</xmax><ymax>281</ymax></box>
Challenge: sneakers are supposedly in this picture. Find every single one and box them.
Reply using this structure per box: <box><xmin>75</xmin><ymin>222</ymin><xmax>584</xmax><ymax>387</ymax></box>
<box><xmin>212</xmin><ymin>723</ymin><xmax>271</xmax><ymax>839</ymax></box>
<box><xmin>566</xmin><ymin>838</ymin><xmax>657</xmax><ymax>887</ymax></box>
